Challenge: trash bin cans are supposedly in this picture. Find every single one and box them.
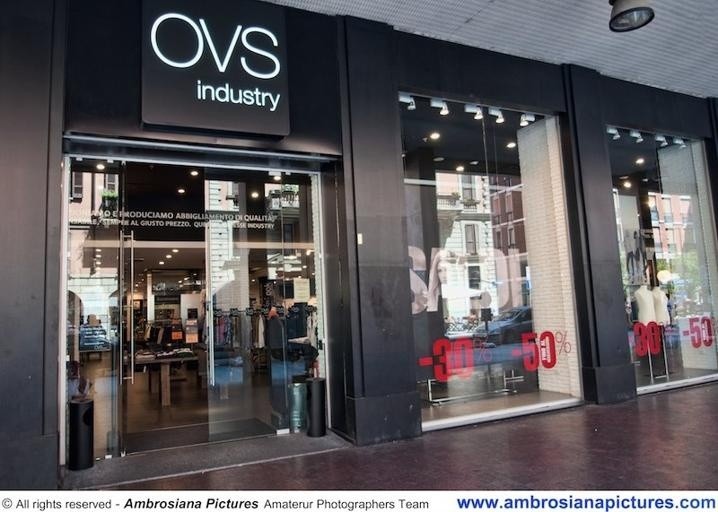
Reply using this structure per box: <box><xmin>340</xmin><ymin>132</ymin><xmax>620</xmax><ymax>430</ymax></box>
<box><xmin>68</xmin><ymin>398</ymin><xmax>94</xmax><ymax>471</ymax></box>
<box><xmin>288</xmin><ymin>377</ymin><xmax>326</xmax><ymax>437</ymax></box>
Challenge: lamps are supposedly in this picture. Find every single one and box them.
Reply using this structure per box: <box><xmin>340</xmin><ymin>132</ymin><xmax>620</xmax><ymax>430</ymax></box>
<box><xmin>399</xmin><ymin>94</ymin><xmax>688</xmax><ymax>147</ymax></box>
<box><xmin>606</xmin><ymin>0</ymin><xmax>655</xmax><ymax>32</ymax></box>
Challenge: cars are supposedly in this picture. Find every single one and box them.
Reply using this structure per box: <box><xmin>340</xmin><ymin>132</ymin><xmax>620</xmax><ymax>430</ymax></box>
<box><xmin>473</xmin><ymin>305</ymin><xmax>535</xmax><ymax>346</ymax></box>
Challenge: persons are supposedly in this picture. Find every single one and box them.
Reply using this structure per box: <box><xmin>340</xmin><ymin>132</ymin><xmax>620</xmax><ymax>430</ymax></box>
<box><xmin>645</xmin><ymin>232</ymin><xmax>656</xmax><ymax>287</ymax></box>
<box><xmin>639</xmin><ymin>230</ymin><xmax>649</xmax><ymax>283</ymax></box>
<box><xmin>651</xmin><ymin>285</ymin><xmax>671</xmax><ymax>327</ymax></box>
<box><xmin>633</xmin><ymin>231</ymin><xmax>643</xmax><ymax>282</ymax></box>
<box><xmin>424</xmin><ymin>247</ymin><xmax>465</xmax><ymax>301</ymax></box>
<box><xmin>634</xmin><ymin>284</ymin><xmax>657</xmax><ymax>327</ymax></box>
<box><xmin>624</xmin><ymin>230</ymin><xmax>635</xmax><ymax>285</ymax></box>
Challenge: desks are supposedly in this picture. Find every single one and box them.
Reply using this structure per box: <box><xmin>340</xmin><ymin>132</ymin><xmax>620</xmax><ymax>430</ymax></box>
<box><xmin>133</xmin><ymin>356</ymin><xmax>199</xmax><ymax>406</ymax></box>
